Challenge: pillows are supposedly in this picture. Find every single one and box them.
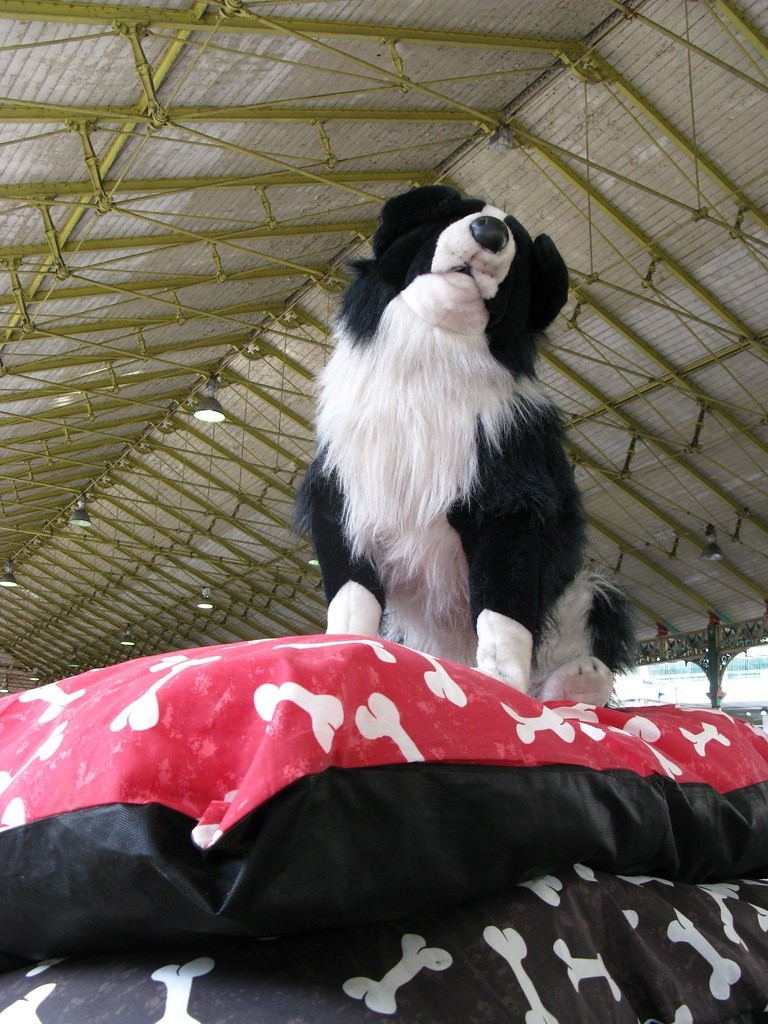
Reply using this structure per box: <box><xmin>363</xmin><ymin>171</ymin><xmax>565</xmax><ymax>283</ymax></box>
<box><xmin>0</xmin><ymin>633</ymin><xmax>768</xmax><ymax>957</ymax></box>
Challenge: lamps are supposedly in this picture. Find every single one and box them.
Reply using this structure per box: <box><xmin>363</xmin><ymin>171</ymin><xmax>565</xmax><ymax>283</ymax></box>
<box><xmin>68</xmin><ymin>493</ymin><xmax>92</xmax><ymax>527</ymax></box>
<box><xmin>198</xmin><ymin>587</ymin><xmax>213</xmax><ymax>609</ymax></box>
<box><xmin>0</xmin><ymin>558</ymin><xmax>17</xmax><ymax>586</ymax></box>
<box><xmin>120</xmin><ymin>624</ymin><xmax>135</xmax><ymax>645</ymax></box>
<box><xmin>699</xmin><ymin>522</ymin><xmax>725</xmax><ymax>561</ymax></box>
<box><xmin>30</xmin><ymin>668</ymin><xmax>40</xmax><ymax>680</ymax></box>
<box><xmin>193</xmin><ymin>373</ymin><xmax>226</xmax><ymax>423</ymax></box>
<box><xmin>0</xmin><ymin>677</ymin><xmax>9</xmax><ymax>692</ymax></box>
<box><xmin>69</xmin><ymin>649</ymin><xmax>80</xmax><ymax>666</ymax></box>
